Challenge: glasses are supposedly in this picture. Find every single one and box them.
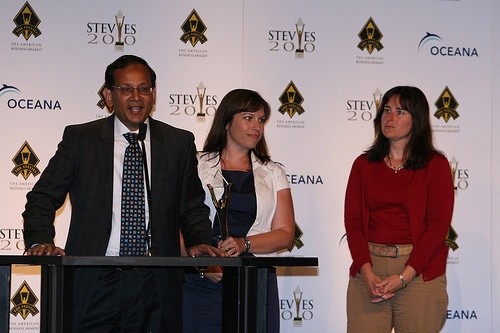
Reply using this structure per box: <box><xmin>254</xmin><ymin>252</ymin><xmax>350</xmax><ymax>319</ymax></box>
<box><xmin>112</xmin><ymin>86</ymin><xmax>153</xmax><ymax>95</ymax></box>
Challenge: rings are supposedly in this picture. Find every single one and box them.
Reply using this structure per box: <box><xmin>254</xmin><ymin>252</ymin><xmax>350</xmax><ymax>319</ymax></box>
<box><xmin>41</xmin><ymin>244</ymin><xmax>45</xmax><ymax>248</ymax></box>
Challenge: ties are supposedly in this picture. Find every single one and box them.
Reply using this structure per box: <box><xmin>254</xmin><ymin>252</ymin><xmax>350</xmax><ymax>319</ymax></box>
<box><xmin>119</xmin><ymin>133</ymin><xmax>146</xmax><ymax>257</ymax></box>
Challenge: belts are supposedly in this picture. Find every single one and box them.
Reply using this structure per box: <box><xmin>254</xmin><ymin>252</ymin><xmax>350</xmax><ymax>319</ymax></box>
<box><xmin>367</xmin><ymin>242</ymin><xmax>413</xmax><ymax>257</ymax></box>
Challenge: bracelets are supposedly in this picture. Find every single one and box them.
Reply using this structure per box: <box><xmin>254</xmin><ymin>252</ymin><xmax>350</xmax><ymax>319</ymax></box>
<box><xmin>398</xmin><ymin>272</ymin><xmax>407</xmax><ymax>289</ymax></box>
<box><xmin>199</xmin><ymin>265</ymin><xmax>206</xmax><ymax>280</ymax></box>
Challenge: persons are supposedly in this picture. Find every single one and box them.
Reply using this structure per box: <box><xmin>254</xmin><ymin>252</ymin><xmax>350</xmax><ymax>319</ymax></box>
<box><xmin>22</xmin><ymin>55</ymin><xmax>229</xmax><ymax>332</ymax></box>
<box><xmin>343</xmin><ymin>85</ymin><xmax>455</xmax><ymax>332</ymax></box>
<box><xmin>179</xmin><ymin>88</ymin><xmax>296</xmax><ymax>333</ymax></box>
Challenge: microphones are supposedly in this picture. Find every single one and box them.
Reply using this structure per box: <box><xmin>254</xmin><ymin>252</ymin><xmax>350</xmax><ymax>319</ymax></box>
<box><xmin>139</xmin><ymin>123</ymin><xmax>153</xmax><ymax>257</ymax></box>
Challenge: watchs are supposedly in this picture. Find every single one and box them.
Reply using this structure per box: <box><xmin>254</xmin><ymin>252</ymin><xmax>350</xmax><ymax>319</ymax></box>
<box><xmin>242</xmin><ymin>236</ymin><xmax>251</xmax><ymax>254</ymax></box>
